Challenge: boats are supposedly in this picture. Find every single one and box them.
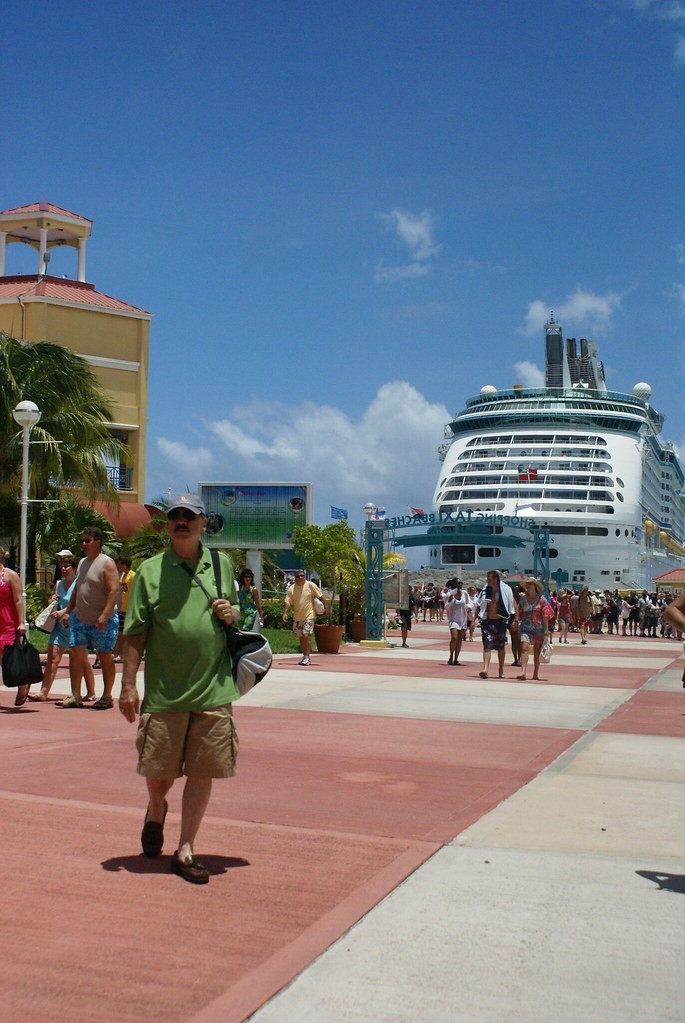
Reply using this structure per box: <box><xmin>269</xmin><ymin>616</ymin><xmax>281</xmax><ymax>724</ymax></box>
<box><xmin>645</xmin><ymin>520</ymin><xmax>658</xmax><ymax>537</ymax></box>
<box><xmin>659</xmin><ymin>530</ymin><xmax>685</xmax><ymax>555</ymax></box>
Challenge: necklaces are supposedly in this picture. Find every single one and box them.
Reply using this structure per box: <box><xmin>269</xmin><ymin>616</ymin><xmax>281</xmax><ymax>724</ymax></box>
<box><xmin>62</xmin><ymin>575</ymin><xmax>76</xmax><ymax>581</ymax></box>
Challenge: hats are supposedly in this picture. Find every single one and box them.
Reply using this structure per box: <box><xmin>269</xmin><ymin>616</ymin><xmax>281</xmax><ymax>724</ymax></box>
<box><xmin>593</xmin><ymin>589</ymin><xmax>602</xmax><ymax>594</ymax></box>
<box><xmin>520</xmin><ymin>576</ymin><xmax>544</xmax><ymax>593</ymax></box>
<box><xmin>581</xmin><ymin>585</ymin><xmax>589</xmax><ymax>592</ymax></box>
<box><xmin>54</xmin><ymin>550</ymin><xmax>72</xmax><ymax>559</ymax></box>
<box><xmin>165</xmin><ymin>494</ymin><xmax>207</xmax><ymax>517</ymax></box>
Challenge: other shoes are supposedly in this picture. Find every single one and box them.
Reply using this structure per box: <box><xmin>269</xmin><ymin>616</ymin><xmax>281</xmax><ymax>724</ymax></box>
<box><xmin>396</xmin><ymin>611</ymin><xmax>684</xmax><ymax>680</ymax></box>
<box><xmin>15</xmin><ymin>650</ymin><xmax>148</xmax><ymax>710</ymax></box>
<box><xmin>141</xmin><ymin>798</ymin><xmax>168</xmax><ymax>858</ymax></box>
<box><xmin>171</xmin><ymin>849</ymin><xmax>210</xmax><ymax>884</ymax></box>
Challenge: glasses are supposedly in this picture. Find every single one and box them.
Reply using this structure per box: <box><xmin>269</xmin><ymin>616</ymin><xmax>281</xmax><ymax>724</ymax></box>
<box><xmin>79</xmin><ymin>539</ymin><xmax>96</xmax><ymax>544</ymax></box>
<box><xmin>60</xmin><ymin>564</ymin><xmax>73</xmax><ymax>569</ymax></box>
<box><xmin>455</xmin><ymin>581</ymin><xmax>463</xmax><ymax>585</ymax></box>
<box><xmin>295</xmin><ymin>574</ymin><xmax>305</xmax><ymax>578</ymax></box>
<box><xmin>166</xmin><ymin>511</ymin><xmax>202</xmax><ymax>522</ymax></box>
<box><xmin>244</xmin><ymin>576</ymin><xmax>252</xmax><ymax>578</ymax></box>
<box><xmin>58</xmin><ymin>556</ymin><xmax>69</xmax><ymax>562</ymax></box>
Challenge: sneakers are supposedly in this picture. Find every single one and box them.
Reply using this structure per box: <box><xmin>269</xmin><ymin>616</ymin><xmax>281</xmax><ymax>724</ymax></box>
<box><xmin>299</xmin><ymin>655</ymin><xmax>312</xmax><ymax>665</ymax></box>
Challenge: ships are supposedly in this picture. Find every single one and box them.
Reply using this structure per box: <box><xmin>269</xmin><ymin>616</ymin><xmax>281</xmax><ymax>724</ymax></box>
<box><xmin>390</xmin><ymin>308</ymin><xmax>685</xmax><ymax>593</ymax></box>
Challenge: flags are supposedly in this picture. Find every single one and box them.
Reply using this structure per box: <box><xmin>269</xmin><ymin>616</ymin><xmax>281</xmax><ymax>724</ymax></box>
<box><xmin>331</xmin><ymin>505</ymin><xmax>348</xmax><ymax>519</ymax></box>
<box><xmin>410</xmin><ymin>505</ymin><xmax>425</xmax><ymax>517</ymax></box>
<box><xmin>519</xmin><ymin>468</ymin><xmax>538</xmax><ymax>481</ymax></box>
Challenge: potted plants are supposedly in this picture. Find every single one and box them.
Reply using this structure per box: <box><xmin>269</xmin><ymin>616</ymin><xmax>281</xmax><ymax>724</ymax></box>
<box><xmin>338</xmin><ymin>560</ymin><xmax>367</xmax><ymax>642</ymax></box>
<box><xmin>289</xmin><ymin>521</ymin><xmax>351</xmax><ymax>653</ymax></box>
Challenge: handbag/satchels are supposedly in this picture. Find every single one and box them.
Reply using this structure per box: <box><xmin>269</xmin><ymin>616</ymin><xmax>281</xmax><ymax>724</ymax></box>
<box><xmin>539</xmin><ymin>635</ymin><xmax>551</xmax><ymax>663</ymax></box>
<box><xmin>35</xmin><ymin>580</ymin><xmax>60</xmax><ymax>633</ymax></box>
<box><xmin>226</xmin><ymin>625</ymin><xmax>273</xmax><ymax>697</ymax></box>
<box><xmin>2</xmin><ymin>631</ymin><xmax>45</xmax><ymax>687</ymax></box>
<box><xmin>306</xmin><ymin>580</ymin><xmax>325</xmax><ymax>615</ymax></box>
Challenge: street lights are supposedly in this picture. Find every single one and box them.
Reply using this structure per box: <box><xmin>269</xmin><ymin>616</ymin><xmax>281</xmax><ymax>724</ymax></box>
<box><xmin>11</xmin><ymin>399</ymin><xmax>43</xmax><ymax>645</ymax></box>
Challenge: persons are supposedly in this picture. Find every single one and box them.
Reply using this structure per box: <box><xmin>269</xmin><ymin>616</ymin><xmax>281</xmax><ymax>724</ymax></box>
<box><xmin>396</xmin><ymin>570</ymin><xmax>685</xmax><ymax>683</ymax></box>
<box><xmin>29</xmin><ymin>531</ymin><xmax>118</xmax><ymax>714</ymax></box>
<box><xmin>91</xmin><ymin>553</ymin><xmax>140</xmax><ymax>670</ymax></box>
<box><xmin>282</xmin><ymin>569</ymin><xmax>330</xmax><ymax>667</ymax></box>
<box><xmin>118</xmin><ymin>493</ymin><xmax>242</xmax><ymax>883</ymax></box>
<box><xmin>0</xmin><ymin>547</ymin><xmax>31</xmax><ymax>706</ymax></box>
<box><xmin>236</xmin><ymin>567</ymin><xmax>264</xmax><ymax>632</ymax></box>
